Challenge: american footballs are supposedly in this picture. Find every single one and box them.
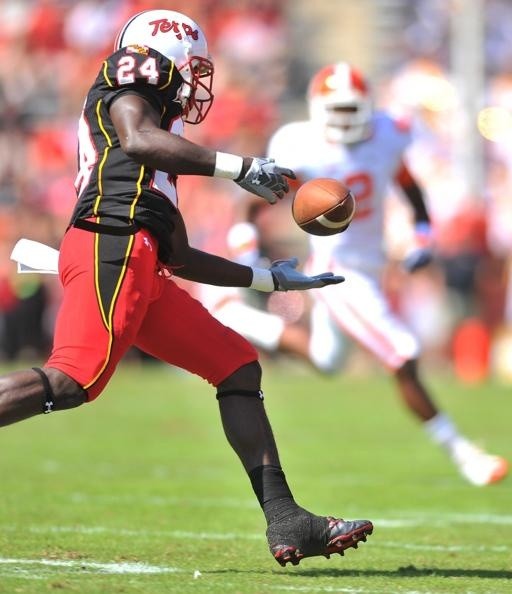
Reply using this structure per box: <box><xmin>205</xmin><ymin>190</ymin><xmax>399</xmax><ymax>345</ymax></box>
<box><xmin>292</xmin><ymin>178</ymin><xmax>356</xmax><ymax>235</ymax></box>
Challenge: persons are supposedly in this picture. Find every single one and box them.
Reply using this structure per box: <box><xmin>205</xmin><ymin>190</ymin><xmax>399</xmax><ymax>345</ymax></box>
<box><xmin>0</xmin><ymin>0</ymin><xmax>512</xmax><ymax>389</ymax></box>
<box><xmin>0</xmin><ymin>8</ymin><xmax>374</xmax><ymax>569</ymax></box>
<box><xmin>204</xmin><ymin>62</ymin><xmax>509</xmax><ymax>491</ymax></box>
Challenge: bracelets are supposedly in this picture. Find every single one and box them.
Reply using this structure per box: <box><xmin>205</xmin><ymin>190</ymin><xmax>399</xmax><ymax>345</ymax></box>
<box><xmin>249</xmin><ymin>265</ymin><xmax>275</xmax><ymax>295</ymax></box>
<box><xmin>213</xmin><ymin>151</ymin><xmax>244</xmax><ymax>181</ymax></box>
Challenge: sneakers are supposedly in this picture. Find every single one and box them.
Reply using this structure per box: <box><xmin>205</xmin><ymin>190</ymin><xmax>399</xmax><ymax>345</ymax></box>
<box><xmin>206</xmin><ymin>284</ymin><xmax>239</xmax><ymax>318</ymax></box>
<box><xmin>450</xmin><ymin>439</ymin><xmax>509</xmax><ymax>485</ymax></box>
<box><xmin>264</xmin><ymin>515</ymin><xmax>375</xmax><ymax>566</ymax></box>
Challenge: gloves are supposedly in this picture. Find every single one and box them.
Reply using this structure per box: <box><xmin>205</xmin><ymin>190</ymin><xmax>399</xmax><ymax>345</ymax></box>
<box><xmin>232</xmin><ymin>153</ymin><xmax>298</xmax><ymax>208</ymax></box>
<box><xmin>226</xmin><ymin>222</ymin><xmax>263</xmax><ymax>266</ymax></box>
<box><xmin>267</xmin><ymin>256</ymin><xmax>345</xmax><ymax>290</ymax></box>
<box><xmin>404</xmin><ymin>225</ymin><xmax>437</xmax><ymax>274</ymax></box>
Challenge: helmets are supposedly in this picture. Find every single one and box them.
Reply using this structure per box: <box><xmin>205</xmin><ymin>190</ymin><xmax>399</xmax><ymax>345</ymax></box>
<box><xmin>307</xmin><ymin>60</ymin><xmax>375</xmax><ymax>145</ymax></box>
<box><xmin>112</xmin><ymin>8</ymin><xmax>210</xmax><ymax>98</ymax></box>
